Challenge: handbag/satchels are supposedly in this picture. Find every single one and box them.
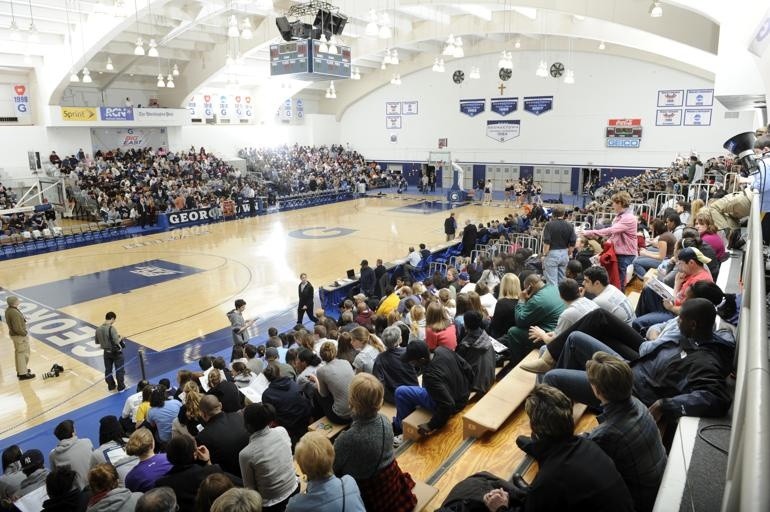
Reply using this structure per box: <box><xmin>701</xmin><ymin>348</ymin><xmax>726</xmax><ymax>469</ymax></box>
<box><xmin>114</xmin><ymin>340</ymin><xmax>126</xmax><ymax>353</ymax></box>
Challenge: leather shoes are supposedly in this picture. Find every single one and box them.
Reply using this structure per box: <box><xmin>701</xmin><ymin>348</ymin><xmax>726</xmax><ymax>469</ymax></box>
<box><xmin>516</xmin><ymin>435</ymin><xmax>535</xmax><ymax>457</ymax></box>
<box><xmin>513</xmin><ymin>472</ymin><xmax>528</xmax><ymax>490</ymax></box>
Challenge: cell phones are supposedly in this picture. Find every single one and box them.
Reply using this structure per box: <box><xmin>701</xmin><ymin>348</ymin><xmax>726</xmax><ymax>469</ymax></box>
<box><xmin>196</xmin><ymin>423</ymin><xmax>205</xmax><ymax>433</ymax></box>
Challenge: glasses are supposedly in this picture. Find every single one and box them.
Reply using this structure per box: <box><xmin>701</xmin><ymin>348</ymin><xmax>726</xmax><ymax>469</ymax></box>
<box><xmin>319</xmin><ymin>423</ymin><xmax>332</xmax><ymax>430</ymax></box>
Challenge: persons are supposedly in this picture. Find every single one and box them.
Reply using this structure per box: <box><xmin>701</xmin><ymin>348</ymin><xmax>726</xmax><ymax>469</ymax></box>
<box><xmin>418</xmin><ymin>172</ymin><xmax>436</xmax><ymax>194</ymax></box>
<box><xmin>478</xmin><ymin>174</ymin><xmax>543</xmax><ymax>209</ymax></box>
<box><xmin>50</xmin><ymin>144</ymin><xmax>399</xmax><ymax>229</ymax></box>
<box><xmin>591</xmin><ymin>125</ymin><xmax>770</xmax><ymax>269</ymax></box>
<box><xmin>125</xmin><ymin>97</ymin><xmax>158</xmax><ymax>108</ymax></box>
<box><xmin>1</xmin><ymin>293</ymin><xmax>404</xmax><ymax>511</ymax></box>
<box><xmin>0</xmin><ymin>186</ymin><xmax>57</xmax><ymax>237</ymax></box>
<box><xmin>297</xmin><ymin>203</ymin><xmax>741</xmax><ymax>511</ymax></box>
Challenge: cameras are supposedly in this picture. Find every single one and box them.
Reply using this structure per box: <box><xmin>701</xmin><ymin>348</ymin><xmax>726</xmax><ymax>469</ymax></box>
<box><xmin>54</xmin><ymin>364</ymin><xmax>64</xmax><ymax>373</ymax></box>
<box><xmin>42</xmin><ymin>370</ymin><xmax>59</xmax><ymax>379</ymax></box>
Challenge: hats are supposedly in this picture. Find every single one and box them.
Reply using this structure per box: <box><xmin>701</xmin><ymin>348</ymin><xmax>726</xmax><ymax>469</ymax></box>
<box><xmin>400</xmin><ymin>341</ymin><xmax>428</xmax><ymax>362</ymax></box>
<box><xmin>20</xmin><ymin>449</ymin><xmax>43</xmax><ymax>470</ymax></box>
<box><xmin>353</xmin><ymin>293</ymin><xmax>366</xmax><ymax>300</ymax></box>
<box><xmin>265</xmin><ymin>347</ymin><xmax>278</xmax><ymax>361</ymax></box>
<box><xmin>678</xmin><ymin>247</ymin><xmax>712</xmax><ymax>264</ymax></box>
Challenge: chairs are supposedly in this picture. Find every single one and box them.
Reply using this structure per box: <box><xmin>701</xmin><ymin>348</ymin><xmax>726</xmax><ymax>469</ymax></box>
<box><xmin>352</xmin><ymin>221</ymin><xmax>530</xmax><ymax>299</ymax></box>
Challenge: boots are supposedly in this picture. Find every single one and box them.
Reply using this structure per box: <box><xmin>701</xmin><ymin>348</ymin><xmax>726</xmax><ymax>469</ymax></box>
<box><xmin>520</xmin><ymin>348</ymin><xmax>555</xmax><ymax>375</ymax></box>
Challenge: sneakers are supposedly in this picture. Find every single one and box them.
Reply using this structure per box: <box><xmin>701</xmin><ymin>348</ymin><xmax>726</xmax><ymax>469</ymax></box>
<box><xmin>117</xmin><ymin>384</ymin><xmax>126</xmax><ymax>392</ymax></box>
<box><xmin>727</xmin><ymin>229</ymin><xmax>746</xmax><ymax>250</ymax></box>
<box><xmin>17</xmin><ymin>369</ymin><xmax>30</xmax><ymax>377</ymax></box>
<box><xmin>20</xmin><ymin>374</ymin><xmax>35</xmax><ymax>379</ymax></box>
<box><xmin>108</xmin><ymin>382</ymin><xmax>116</xmax><ymax>390</ymax></box>
